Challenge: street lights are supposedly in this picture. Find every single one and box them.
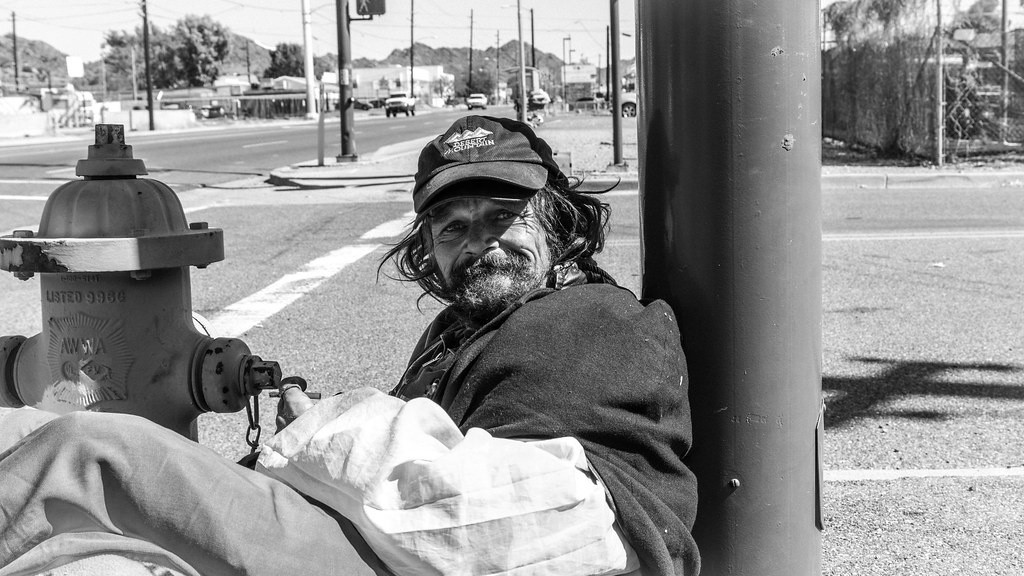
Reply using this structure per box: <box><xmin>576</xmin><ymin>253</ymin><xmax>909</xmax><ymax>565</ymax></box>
<box><xmin>501</xmin><ymin>4</ymin><xmax>535</xmax><ymax>67</ymax></box>
<box><xmin>563</xmin><ymin>37</ymin><xmax>571</xmax><ymax>104</ymax></box>
<box><xmin>568</xmin><ymin>49</ymin><xmax>576</xmax><ymax>85</ymax></box>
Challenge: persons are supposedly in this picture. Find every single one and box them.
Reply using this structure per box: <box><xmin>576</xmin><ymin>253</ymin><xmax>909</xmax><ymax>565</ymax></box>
<box><xmin>0</xmin><ymin>114</ymin><xmax>702</xmax><ymax>576</ymax></box>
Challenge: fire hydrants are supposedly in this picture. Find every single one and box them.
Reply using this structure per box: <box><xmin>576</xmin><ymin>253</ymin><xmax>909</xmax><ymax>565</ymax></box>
<box><xmin>0</xmin><ymin>120</ymin><xmax>282</xmax><ymax>446</ymax></box>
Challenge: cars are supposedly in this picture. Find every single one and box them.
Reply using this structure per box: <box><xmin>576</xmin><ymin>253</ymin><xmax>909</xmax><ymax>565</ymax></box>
<box><xmin>466</xmin><ymin>93</ymin><xmax>488</xmax><ymax>110</ymax></box>
<box><xmin>569</xmin><ymin>96</ymin><xmax>606</xmax><ymax>112</ymax></box>
<box><xmin>608</xmin><ymin>91</ymin><xmax>636</xmax><ymax>118</ymax></box>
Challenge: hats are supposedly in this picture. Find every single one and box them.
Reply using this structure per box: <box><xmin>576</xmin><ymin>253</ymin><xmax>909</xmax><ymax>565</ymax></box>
<box><xmin>413</xmin><ymin>114</ymin><xmax>568</xmax><ymax>213</ymax></box>
<box><xmin>413</xmin><ymin>179</ymin><xmax>538</xmax><ymax>230</ymax></box>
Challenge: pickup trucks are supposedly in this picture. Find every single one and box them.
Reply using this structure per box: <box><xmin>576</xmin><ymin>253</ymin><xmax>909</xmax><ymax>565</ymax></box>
<box><xmin>385</xmin><ymin>92</ymin><xmax>417</xmax><ymax>118</ymax></box>
